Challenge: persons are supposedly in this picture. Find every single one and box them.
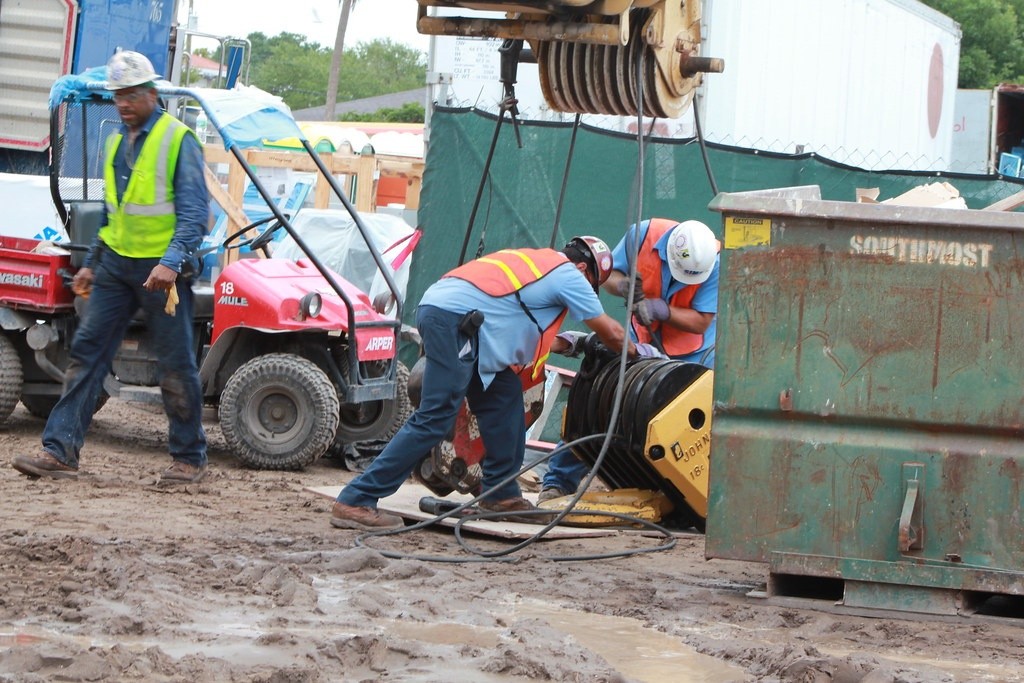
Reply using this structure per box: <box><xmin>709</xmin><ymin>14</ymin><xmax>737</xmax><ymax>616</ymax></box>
<box><xmin>9</xmin><ymin>51</ymin><xmax>209</xmax><ymax>486</ymax></box>
<box><xmin>331</xmin><ymin>236</ymin><xmax>670</xmax><ymax>533</ymax></box>
<box><xmin>538</xmin><ymin>217</ymin><xmax>724</xmax><ymax>505</ymax></box>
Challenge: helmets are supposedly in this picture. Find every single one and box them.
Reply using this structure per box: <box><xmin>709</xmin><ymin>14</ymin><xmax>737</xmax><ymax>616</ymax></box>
<box><xmin>105</xmin><ymin>50</ymin><xmax>162</xmax><ymax>90</ymax></box>
<box><xmin>571</xmin><ymin>234</ymin><xmax>613</xmax><ymax>297</ymax></box>
<box><xmin>667</xmin><ymin>220</ymin><xmax>718</xmax><ymax>284</ymax></box>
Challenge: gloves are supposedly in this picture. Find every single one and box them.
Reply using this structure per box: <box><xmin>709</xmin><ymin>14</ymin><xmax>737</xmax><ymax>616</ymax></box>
<box><xmin>629</xmin><ymin>297</ymin><xmax>670</xmax><ymax>327</ymax></box>
<box><xmin>554</xmin><ymin>330</ymin><xmax>589</xmax><ymax>356</ymax></box>
<box><xmin>620</xmin><ymin>274</ymin><xmax>646</xmax><ymax>303</ymax></box>
<box><xmin>629</xmin><ymin>343</ymin><xmax>672</xmax><ymax>365</ymax></box>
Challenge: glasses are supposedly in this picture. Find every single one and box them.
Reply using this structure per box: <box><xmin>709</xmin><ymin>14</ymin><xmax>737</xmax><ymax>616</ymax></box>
<box><xmin>113</xmin><ymin>89</ymin><xmax>146</xmax><ymax>103</ymax></box>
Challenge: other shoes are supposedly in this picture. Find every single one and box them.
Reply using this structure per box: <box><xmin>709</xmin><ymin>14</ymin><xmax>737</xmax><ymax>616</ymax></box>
<box><xmin>331</xmin><ymin>500</ymin><xmax>404</xmax><ymax>531</ymax></box>
<box><xmin>159</xmin><ymin>458</ymin><xmax>210</xmax><ymax>485</ymax></box>
<box><xmin>11</xmin><ymin>451</ymin><xmax>78</xmax><ymax>481</ymax></box>
<box><xmin>536</xmin><ymin>486</ymin><xmax>565</xmax><ymax>506</ymax></box>
<box><xmin>479</xmin><ymin>497</ymin><xmax>555</xmax><ymax>525</ymax></box>
